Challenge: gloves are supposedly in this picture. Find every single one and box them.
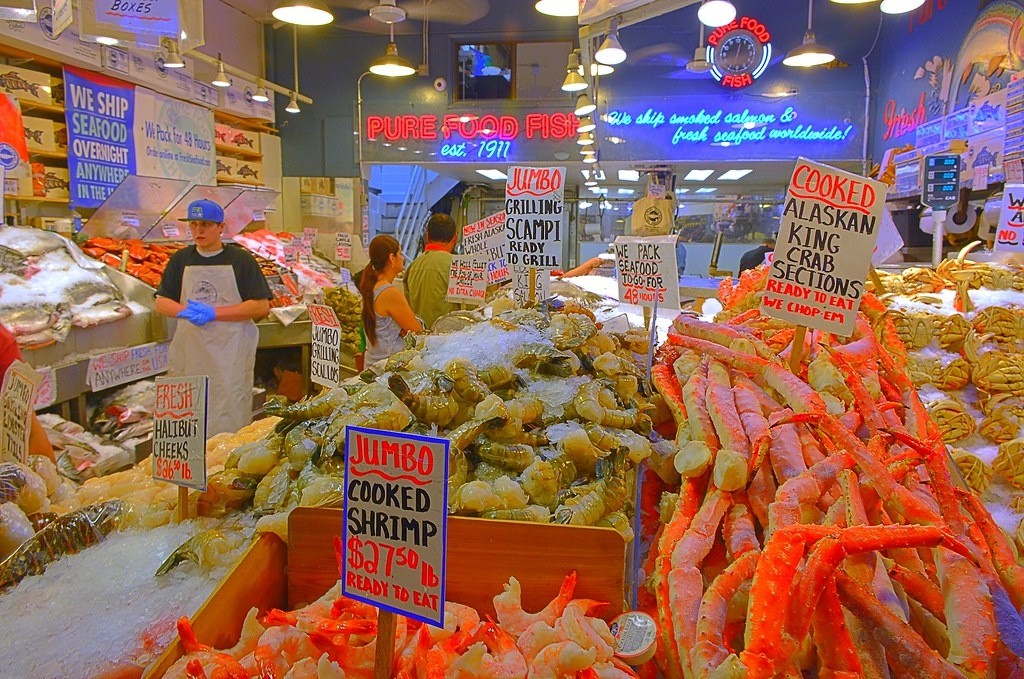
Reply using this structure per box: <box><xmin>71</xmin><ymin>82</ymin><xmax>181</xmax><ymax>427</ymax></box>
<box><xmin>177</xmin><ymin>299</ymin><xmax>215</xmax><ymax>326</ymax></box>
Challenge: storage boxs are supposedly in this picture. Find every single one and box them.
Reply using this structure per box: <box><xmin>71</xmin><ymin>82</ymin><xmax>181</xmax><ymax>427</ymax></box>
<box><xmin>0</xmin><ymin>64</ymin><xmax>263</xmax><ymax>199</ymax></box>
<box><xmin>300</xmin><ymin>194</ymin><xmax>339</xmax><ymax>218</ymax></box>
<box><xmin>301</xmin><ymin>177</ymin><xmax>330</xmax><ymax>194</ymax></box>
<box><xmin>143</xmin><ymin>505</ymin><xmax>625</xmax><ymax>679</ymax></box>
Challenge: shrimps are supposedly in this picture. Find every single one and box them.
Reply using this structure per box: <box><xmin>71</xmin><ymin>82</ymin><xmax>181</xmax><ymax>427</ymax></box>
<box><xmin>0</xmin><ymin>305</ymin><xmax>657</xmax><ymax>593</ymax></box>
<box><xmin>173</xmin><ymin>576</ymin><xmax>639</xmax><ymax>679</ymax></box>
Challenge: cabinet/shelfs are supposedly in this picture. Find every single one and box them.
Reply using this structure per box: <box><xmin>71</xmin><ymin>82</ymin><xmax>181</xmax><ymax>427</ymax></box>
<box><xmin>0</xmin><ymin>33</ymin><xmax>279</xmax><ymax>204</ymax></box>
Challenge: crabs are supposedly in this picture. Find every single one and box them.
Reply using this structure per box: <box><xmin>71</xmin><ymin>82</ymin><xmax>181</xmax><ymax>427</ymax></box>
<box><xmin>82</xmin><ymin>235</ymin><xmax>188</xmax><ymax>287</ymax></box>
<box><xmin>649</xmin><ymin>265</ymin><xmax>1023</xmax><ymax>679</ymax></box>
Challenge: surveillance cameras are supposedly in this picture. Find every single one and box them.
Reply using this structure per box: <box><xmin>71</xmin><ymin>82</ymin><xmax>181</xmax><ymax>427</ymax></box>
<box><xmin>433</xmin><ymin>77</ymin><xmax>446</xmax><ymax>90</ymax></box>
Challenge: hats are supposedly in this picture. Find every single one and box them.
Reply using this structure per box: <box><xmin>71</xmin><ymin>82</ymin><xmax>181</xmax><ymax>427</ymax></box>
<box><xmin>177</xmin><ymin>199</ymin><xmax>224</xmax><ymax>222</ymax></box>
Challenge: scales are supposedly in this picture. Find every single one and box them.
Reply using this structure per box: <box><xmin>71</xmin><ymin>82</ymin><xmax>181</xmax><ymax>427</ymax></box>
<box><xmin>877</xmin><ymin>152</ymin><xmax>962</xmax><ymax>273</ymax></box>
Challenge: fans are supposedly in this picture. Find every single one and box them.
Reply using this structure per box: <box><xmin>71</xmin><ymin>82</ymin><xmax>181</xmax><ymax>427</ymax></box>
<box><xmin>328</xmin><ymin>0</ymin><xmax>492</xmax><ymax>25</ymax></box>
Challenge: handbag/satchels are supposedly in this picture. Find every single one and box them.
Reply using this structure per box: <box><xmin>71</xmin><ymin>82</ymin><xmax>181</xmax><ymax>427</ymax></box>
<box><xmin>352</xmin><ymin>349</ymin><xmax>368</xmax><ymax>372</ymax></box>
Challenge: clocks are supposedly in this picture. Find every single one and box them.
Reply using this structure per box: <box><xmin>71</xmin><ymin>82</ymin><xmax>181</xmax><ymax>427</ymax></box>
<box><xmin>707</xmin><ymin>18</ymin><xmax>772</xmax><ymax>87</ymax></box>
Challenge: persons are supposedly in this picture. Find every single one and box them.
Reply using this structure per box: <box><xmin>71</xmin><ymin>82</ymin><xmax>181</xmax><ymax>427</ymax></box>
<box><xmin>0</xmin><ymin>325</ymin><xmax>60</xmax><ymax>477</ymax></box>
<box><xmin>153</xmin><ymin>198</ymin><xmax>273</xmax><ymax>441</ymax></box>
<box><xmin>403</xmin><ymin>215</ymin><xmax>479</xmax><ymax>331</ymax></box>
<box><xmin>361</xmin><ymin>235</ymin><xmax>422</xmax><ymax>370</ymax></box>
<box><xmin>676</xmin><ymin>238</ymin><xmax>774</xmax><ymax>284</ymax></box>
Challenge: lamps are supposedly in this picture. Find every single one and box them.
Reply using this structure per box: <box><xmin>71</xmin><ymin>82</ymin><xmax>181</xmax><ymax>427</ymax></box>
<box><xmin>576</xmin><ymin>116</ymin><xmax>596</xmax><ymax>133</ymax></box>
<box><xmin>583</xmin><ymin>155</ymin><xmax>597</xmax><ymax>163</ymax></box>
<box><xmin>211</xmin><ymin>61</ymin><xmax>231</xmax><ymax>88</ymax></box>
<box><xmin>573</xmin><ymin>91</ymin><xmax>597</xmax><ymax>115</ymax></box>
<box><xmin>782</xmin><ymin>0</ymin><xmax>836</xmax><ymax>69</ymax></box>
<box><xmin>370</xmin><ymin>23</ymin><xmax>418</xmax><ymax>78</ymax></box>
<box><xmin>594</xmin><ymin>12</ymin><xmax>626</xmax><ymax>66</ymax></box>
<box><xmin>285</xmin><ymin>92</ymin><xmax>300</xmax><ymax>114</ymax></box>
<box><xmin>582</xmin><ymin>170</ymin><xmax>600</xmax><ymax>191</ymax></box>
<box><xmin>271</xmin><ymin>0</ymin><xmax>335</xmax><ymax>26</ymax></box>
<box><xmin>253</xmin><ymin>80</ymin><xmax>270</xmax><ymax>103</ymax></box>
<box><xmin>562</xmin><ymin>47</ymin><xmax>589</xmax><ymax>91</ymax></box>
<box><xmin>162</xmin><ymin>40</ymin><xmax>184</xmax><ymax>67</ymax></box>
<box><xmin>580</xmin><ymin>145</ymin><xmax>596</xmax><ymax>155</ymax></box>
<box><xmin>577</xmin><ymin>132</ymin><xmax>596</xmax><ymax>146</ymax></box>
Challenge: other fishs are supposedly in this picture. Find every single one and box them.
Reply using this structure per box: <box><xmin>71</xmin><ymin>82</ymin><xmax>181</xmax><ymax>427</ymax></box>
<box><xmin>92</xmin><ymin>388</ymin><xmax>155</xmax><ymax>444</ymax></box>
<box><xmin>0</xmin><ymin>224</ymin><xmax>133</xmax><ymax>349</ymax></box>
